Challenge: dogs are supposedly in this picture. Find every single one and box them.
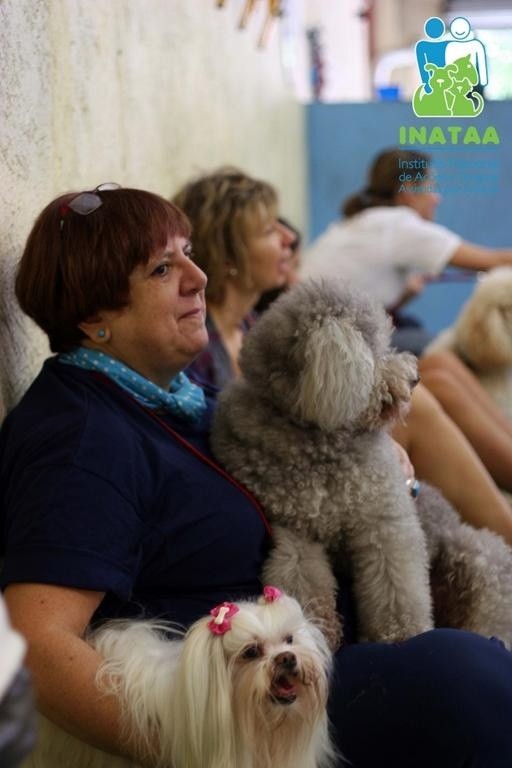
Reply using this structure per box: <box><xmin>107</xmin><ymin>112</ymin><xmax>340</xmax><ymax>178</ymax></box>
<box><xmin>208</xmin><ymin>264</ymin><xmax>511</xmax><ymax>655</ymax></box>
<box><xmin>19</xmin><ymin>586</ymin><xmax>347</xmax><ymax>768</ymax></box>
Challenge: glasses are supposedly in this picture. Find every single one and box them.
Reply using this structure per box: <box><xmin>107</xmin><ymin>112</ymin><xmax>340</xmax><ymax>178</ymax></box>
<box><xmin>64</xmin><ymin>180</ymin><xmax>123</xmax><ymax>218</ymax></box>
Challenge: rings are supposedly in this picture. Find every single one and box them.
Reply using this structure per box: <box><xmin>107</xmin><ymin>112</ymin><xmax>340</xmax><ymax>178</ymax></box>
<box><xmin>403</xmin><ymin>476</ymin><xmax>415</xmax><ymax>487</ymax></box>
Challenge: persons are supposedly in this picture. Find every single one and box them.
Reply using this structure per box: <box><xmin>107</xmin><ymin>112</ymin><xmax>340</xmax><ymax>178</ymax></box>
<box><xmin>0</xmin><ymin>185</ymin><xmax>512</xmax><ymax>768</ymax></box>
<box><xmin>296</xmin><ymin>148</ymin><xmax>512</xmax><ymax>356</ymax></box>
<box><xmin>172</xmin><ymin>165</ymin><xmax>512</xmax><ymax>550</ymax></box>
<box><xmin>251</xmin><ymin>214</ymin><xmax>512</xmax><ymax>494</ymax></box>
